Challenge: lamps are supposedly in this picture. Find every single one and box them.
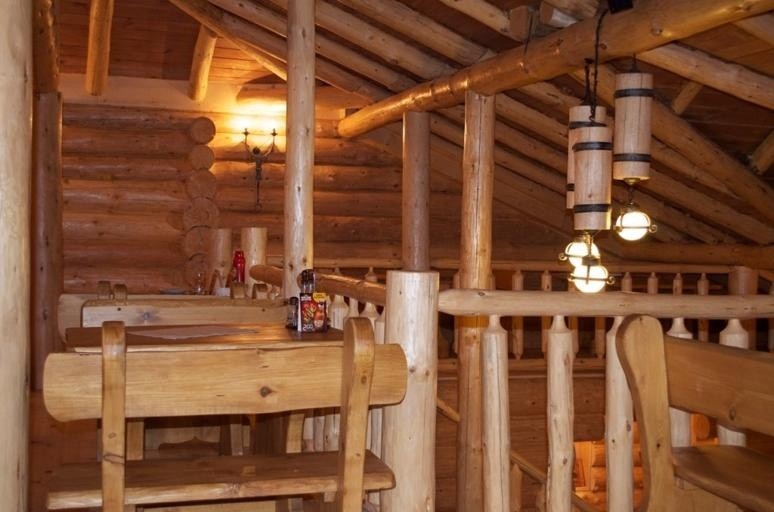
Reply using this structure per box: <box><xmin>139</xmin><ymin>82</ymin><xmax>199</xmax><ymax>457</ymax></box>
<box><xmin>244</xmin><ymin>127</ymin><xmax>277</xmax><ymax>209</ymax></box>
<box><xmin>558</xmin><ymin>14</ymin><xmax>657</xmax><ymax>294</ymax></box>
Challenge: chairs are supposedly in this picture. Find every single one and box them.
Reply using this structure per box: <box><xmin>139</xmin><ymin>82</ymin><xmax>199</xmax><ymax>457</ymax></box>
<box><xmin>42</xmin><ymin>279</ymin><xmax>408</xmax><ymax>512</ymax></box>
<box><xmin>618</xmin><ymin>314</ymin><xmax>774</xmax><ymax>511</ymax></box>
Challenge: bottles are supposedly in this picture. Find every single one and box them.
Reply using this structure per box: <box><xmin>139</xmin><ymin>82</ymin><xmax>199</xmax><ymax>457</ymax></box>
<box><xmin>285</xmin><ymin>268</ymin><xmax>316</xmax><ymax>331</ymax></box>
<box><xmin>229</xmin><ymin>249</ymin><xmax>244</xmax><ymax>286</ymax></box>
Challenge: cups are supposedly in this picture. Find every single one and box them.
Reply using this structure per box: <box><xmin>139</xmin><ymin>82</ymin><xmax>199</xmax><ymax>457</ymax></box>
<box><xmin>96</xmin><ymin>280</ymin><xmax>127</xmax><ymax>301</ymax></box>
